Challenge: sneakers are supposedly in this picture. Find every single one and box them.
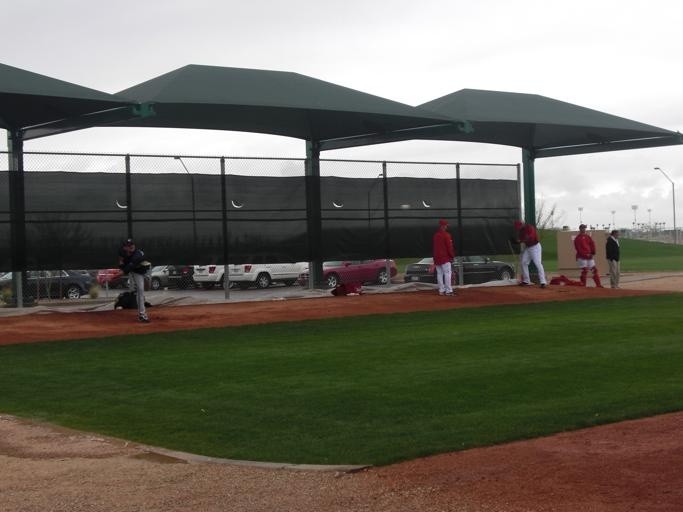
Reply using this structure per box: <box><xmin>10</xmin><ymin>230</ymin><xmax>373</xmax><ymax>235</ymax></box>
<box><xmin>520</xmin><ymin>281</ymin><xmax>531</xmax><ymax>286</ymax></box>
<box><xmin>138</xmin><ymin>313</ymin><xmax>147</xmax><ymax>321</ymax></box>
<box><xmin>447</xmin><ymin>292</ymin><xmax>458</xmax><ymax>296</ymax></box>
<box><xmin>540</xmin><ymin>283</ymin><xmax>547</xmax><ymax>288</ymax></box>
<box><xmin>438</xmin><ymin>291</ymin><xmax>446</xmax><ymax>295</ymax></box>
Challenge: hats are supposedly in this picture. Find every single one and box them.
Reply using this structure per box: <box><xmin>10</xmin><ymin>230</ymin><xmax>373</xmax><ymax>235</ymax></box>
<box><xmin>514</xmin><ymin>220</ymin><xmax>522</xmax><ymax>231</ymax></box>
<box><xmin>126</xmin><ymin>239</ymin><xmax>134</xmax><ymax>245</ymax></box>
<box><xmin>578</xmin><ymin>224</ymin><xmax>586</xmax><ymax>228</ymax></box>
<box><xmin>438</xmin><ymin>219</ymin><xmax>449</xmax><ymax>225</ymax></box>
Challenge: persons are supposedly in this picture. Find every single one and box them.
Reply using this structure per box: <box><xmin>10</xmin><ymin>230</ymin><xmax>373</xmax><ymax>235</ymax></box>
<box><xmin>118</xmin><ymin>239</ymin><xmax>151</xmax><ymax>323</ymax></box>
<box><xmin>604</xmin><ymin>229</ymin><xmax>621</xmax><ymax>288</ymax></box>
<box><xmin>432</xmin><ymin>220</ymin><xmax>455</xmax><ymax>295</ymax></box>
<box><xmin>573</xmin><ymin>224</ymin><xmax>602</xmax><ymax>288</ymax></box>
<box><xmin>512</xmin><ymin>220</ymin><xmax>546</xmax><ymax>288</ymax></box>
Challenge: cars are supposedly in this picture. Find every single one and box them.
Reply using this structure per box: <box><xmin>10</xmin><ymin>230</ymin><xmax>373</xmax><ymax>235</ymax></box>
<box><xmin>97</xmin><ymin>265</ymin><xmax>309</xmax><ymax>289</ymax></box>
<box><xmin>0</xmin><ymin>270</ymin><xmax>92</xmax><ymax>299</ymax></box>
<box><xmin>298</xmin><ymin>258</ymin><xmax>397</xmax><ymax>289</ymax></box>
<box><xmin>403</xmin><ymin>256</ymin><xmax>515</xmax><ymax>286</ymax></box>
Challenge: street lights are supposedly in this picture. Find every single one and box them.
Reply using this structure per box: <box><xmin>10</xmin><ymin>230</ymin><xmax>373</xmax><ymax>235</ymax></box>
<box><xmin>654</xmin><ymin>168</ymin><xmax>675</xmax><ymax>230</ymax></box>
<box><xmin>174</xmin><ymin>157</ymin><xmax>189</xmax><ymax>173</ymax></box>
